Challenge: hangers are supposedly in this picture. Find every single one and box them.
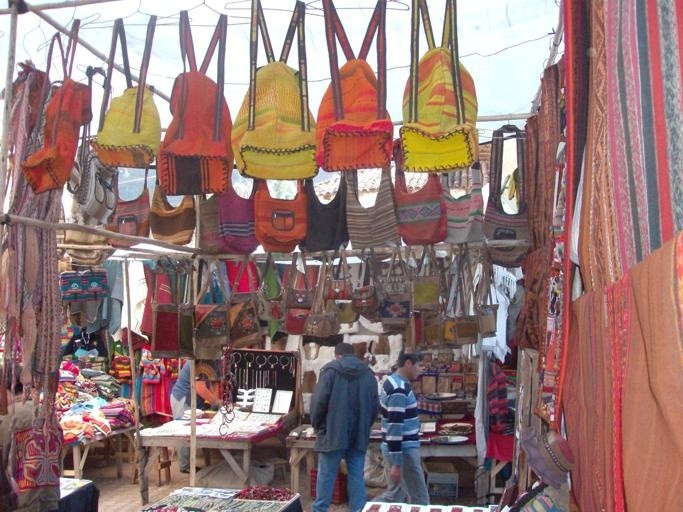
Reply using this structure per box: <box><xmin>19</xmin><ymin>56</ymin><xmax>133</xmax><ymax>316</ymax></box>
<box><xmin>475</xmin><ymin>114</ymin><xmax>529</xmax><ymax>140</ymax></box>
<box><xmin>80</xmin><ymin>0</ymin><xmax>192</xmax><ymax>31</ymax></box>
<box><xmin>76</xmin><ymin>55</ymin><xmax>111</xmax><ymax>89</ymax></box>
<box><xmin>157</xmin><ymin>0</ymin><xmax>252</xmax><ymax>28</ymax></box>
<box><xmin>222</xmin><ymin>0</ymin><xmax>320</xmax><ymax>23</ymax></box>
<box><xmin>33</xmin><ymin>0</ymin><xmax>103</xmax><ymax>54</ymax></box>
<box><xmin>299</xmin><ymin>0</ymin><xmax>412</xmax><ymax>15</ymax></box>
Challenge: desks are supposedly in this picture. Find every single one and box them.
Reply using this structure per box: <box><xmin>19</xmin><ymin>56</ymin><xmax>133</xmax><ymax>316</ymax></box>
<box><xmin>407</xmin><ymin>398</ymin><xmax>472</xmax><ymax>420</ymax></box>
<box><xmin>57</xmin><ymin>473</ymin><xmax>100</xmax><ymax>512</ymax></box>
<box><xmin>133</xmin><ymin>407</ymin><xmax>294</xmax><ymax>501</ymax></box>
<box><xmin>61</xmin><ymin>423</ymin><xmax>145</xmax><ymax>487</ymax></box>
<box><xmin>357</xmin><ymin>502</ymin><xmax>510</xmax><ymax>512</ymax></box>
<box><xmin>140</xmin><ymin>486</ymin><xmax>308</xmax><ymax>512</ymax></box>
<box><xmin>283</xmin><ymin>419</ymin><xmax>480</xmax><ymax>504</ymax></box>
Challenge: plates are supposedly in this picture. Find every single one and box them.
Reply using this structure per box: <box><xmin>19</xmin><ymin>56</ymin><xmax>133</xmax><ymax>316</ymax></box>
<box><xmin>183</xmin><ymin>409</ymin><xmax>201</xmax><ymax>419</ymax></box>
<box><xmin>423</xmin><ymin>391</ymin><xmax>457</xmax><ymax>401</ymax></box>
<box><xmin>429</xmin><ymin>423</ymin><xmax>473</xmax><ymax>444</ymax></box>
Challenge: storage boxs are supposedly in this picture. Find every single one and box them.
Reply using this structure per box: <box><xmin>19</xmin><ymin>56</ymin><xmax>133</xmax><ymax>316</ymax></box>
<box><xmin>422</xmin><ymin>459</ymin><xmax>461</xmax><ymax>499</ymax></box>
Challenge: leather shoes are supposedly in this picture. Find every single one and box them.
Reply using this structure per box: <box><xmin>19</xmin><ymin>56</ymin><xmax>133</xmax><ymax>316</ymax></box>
<box><xmin>182</xmin><ymin>467</ymin><xmax>200</xmax><ymax>474</ymax></box>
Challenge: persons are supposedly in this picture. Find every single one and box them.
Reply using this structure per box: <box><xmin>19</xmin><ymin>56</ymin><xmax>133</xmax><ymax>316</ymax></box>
<box><xmin>308</xmin><ymin>344</ymin><xmax>378</xmax><ymax>512</ymax></box>
<box><xmin>371</xmin><ymin>349</ymin><xmax>431</xmax><ymax>505</ymax></box>
<box><xmin>171</xmin><ymin>359</ymin><xmax>211</xmax><ymax>474</ymax></box>
<box><xmin>516</xmin><ymin>427</ymin><xmax>575</xmax><ymax>512</ymax></box>
<box><xmin>0</xmin><ymin>363</ymin><xmax>62</xmax><ymax>512</ymax></box>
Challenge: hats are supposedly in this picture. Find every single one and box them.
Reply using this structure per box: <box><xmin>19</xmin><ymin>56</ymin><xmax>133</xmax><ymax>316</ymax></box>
<box><xmin>520</xmin><ymin>424</ymin><xmax>576</xmax><ymax>490</ymax></box>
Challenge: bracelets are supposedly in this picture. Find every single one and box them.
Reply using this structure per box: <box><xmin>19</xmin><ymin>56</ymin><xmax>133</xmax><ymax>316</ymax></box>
<box><xmin>219</xmin><ymin>350</ymin><xmax>289</xmax><ymax>437</ymax></box>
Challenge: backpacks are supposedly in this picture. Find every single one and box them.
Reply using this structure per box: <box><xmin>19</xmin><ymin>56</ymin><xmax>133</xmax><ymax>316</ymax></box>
<box><xmin>90</xmin><ymin>14</ymin><xmax>162</xmax><ymax>170</ymax></box>
<box><xmin>399</xmin><ymin>2</ymin><xmax>481</xmax><ymax>174</ymax></box>
<box><xmin>233</xmin><ymin>5</ymin><xmax>319</xmax><ymax>183</ymax></box>
<box><xmin>154</xmin><ymin>11</ymin><xmax>235</xmax><ymax>198</ymax></box>
<box><xmin>23</xmin><ymin>23</ymin><xmax>93</xmax><ymax>195</ymax></box>
<box><xmin>312</xmin><ymin>0</ymin><xmax>396</xmax><ymax>172</ymax></box>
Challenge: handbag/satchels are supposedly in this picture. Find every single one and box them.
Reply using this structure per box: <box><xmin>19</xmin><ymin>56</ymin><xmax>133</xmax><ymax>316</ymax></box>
<box><xmin>54</xmin><ymin>142</ymin><xmax>152</xmax><ymax>267</ymax></box>
<box><xmin>215</xmin><ymin>181</ymin><xmax>264</xmax><ymax>253</ymax></box>
<box><xmin>197</xmin><ymin>192</ymin><xmax>223</xmax><ymax>254</ymax></box>
<box><xmin>142</xmin><ymin>245</ymin><xmax>499</xmax><ymax>381</ymax></box>
<box><xmin>388</xmin><ymin>154</ymin><xmax>448</xmax><ymax>248</ymax></box>
<box><xmin>344</xmin><ymin>167</ymin><xmax>403</xmax><ymax>251</ymax></box>
<box><xmin>256</xmin><ymin>176</ymin><xmax>307</xmax><ymax>254</ymax></box>
<box><xmin>482</xmin><ymin>123</ymin><xmax>534</xmax><ymax>268</ymax></box>
<box><xmin>149</xmin><ymin>156</ymin><xmax>196</xmax><ymax>245</ymax></box>
<box><xmin>302</xmin><ymin>175</ymin><xmax>348</xmax><ymax>255</ymax></box>
<box><xmin>440</xmin><ymin>165</ymin><xmax>485</xmax><ymax>247</ymax></box>
